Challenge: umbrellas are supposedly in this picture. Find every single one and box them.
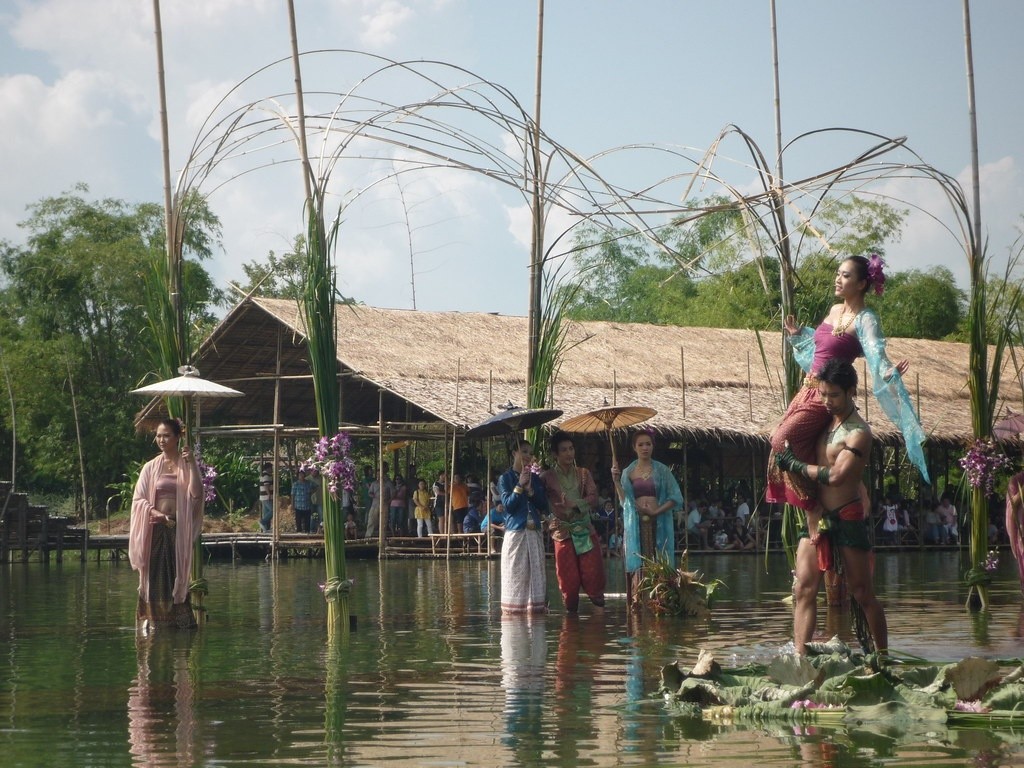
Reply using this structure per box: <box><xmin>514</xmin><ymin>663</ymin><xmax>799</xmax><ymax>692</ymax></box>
<box><xmin>464</xmin><ymin>400</ymin><xmax>565</xmax><ymax>476</ymax></box>
<box><xmin>128</xmin><ymin>366</ymin><xmax>247</xmax><ymax>451</ymax></box>
<box><xmin>560</xmin><ymin>398</ymin><xmax>657</xmax><ymax>476</ymax></box>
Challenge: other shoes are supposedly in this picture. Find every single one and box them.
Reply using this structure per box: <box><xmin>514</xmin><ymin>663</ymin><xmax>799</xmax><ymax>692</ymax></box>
<box><xmin>258</xmin><ymin>519</ymin><xmax>268</xmax><ymax>531</ymax></box>
<box><xmin>491</xmin><ymin>549</ymin><xmax>496</xmax><ymax>554</ymax></box>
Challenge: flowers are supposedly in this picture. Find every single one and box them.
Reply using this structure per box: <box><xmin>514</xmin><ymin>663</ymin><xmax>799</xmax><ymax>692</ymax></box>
<box><xmin>958</xmin><ymin>437</ymin><xmax>1014</xmax><ymax>499</ymax></box>
<box><xmin>527</xmin><ymin>460</ymin><xmax>542</xmax><ymax>476</ymax></box>
<box><xmin>194</xmin><ymin>428</ymin><xmax>216</xmax><ymax>501</ymax></box>
<box><xmin>301</xmin><ymin>430</ymin><xmax>363</xmax><ymax>499</ymax></box>
<box><xmin>981</xmin><ymin>545</ymin><xmax>1001</xmax><ymax>573</ymax></box>
<box><xmin>318</xmin><ymin>577</ymin><xmax>357</xmax><ymax>602</ymax></box>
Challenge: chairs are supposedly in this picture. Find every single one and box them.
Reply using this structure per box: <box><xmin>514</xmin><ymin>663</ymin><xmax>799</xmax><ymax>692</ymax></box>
<box><xmin>541</xmin><ymin>503</ymin><xmax>925</xmax><ymax>549</ymax></box>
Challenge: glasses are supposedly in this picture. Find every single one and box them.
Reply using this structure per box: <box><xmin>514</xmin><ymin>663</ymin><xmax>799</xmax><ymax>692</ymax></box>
<box><xmin>396</xmin><ymin>478</ymin><xmax>402</xmax><ymax>481</ymax></box>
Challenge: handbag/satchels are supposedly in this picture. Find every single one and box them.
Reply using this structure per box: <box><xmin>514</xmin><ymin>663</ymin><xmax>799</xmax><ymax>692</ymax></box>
<box><xmin>569</xmin><ymin>518</ymin><xmax>595</xmax><ymax>556</ymax></box>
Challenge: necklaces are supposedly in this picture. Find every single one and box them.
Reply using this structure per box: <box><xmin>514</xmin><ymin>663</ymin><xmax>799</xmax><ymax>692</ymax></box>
<box><xmin>831</xmin><ymin>307</ymin><xmax>859</xmax><ymax>337</ymax></box>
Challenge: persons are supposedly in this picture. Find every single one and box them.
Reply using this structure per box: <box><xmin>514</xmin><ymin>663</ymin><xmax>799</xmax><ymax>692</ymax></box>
<box><xmin>774</xmin><ymin>360</ymin><xmax>894</xmax><ymax>659</ymax></box>
<box><xmin>256</xmin><ymin>441</ymin><xmax>1013</xmax><ymax>550</ymax></box>
<box><xmin>496</xmin><ymin>439</ymin><xmax>551</xmax><ymax>616</ymax></box>
<box><xmin>768</xmin><ymin>252</ymin><xmax>912</xmax><ymax>501</ymax></box>
<box><xmin>542</xmin><ymin>432</ymin><xmax>608</xmax><ymax>615</ymax></box>
<box><xmin>609</xmin><ymin>429</ymin><xmax>685</xmax><ymax>614</ymax></box>
<box><xmin>127</xmin><ymin>419</ymin><xmax>206</xmax><ymax>632</ymax></box>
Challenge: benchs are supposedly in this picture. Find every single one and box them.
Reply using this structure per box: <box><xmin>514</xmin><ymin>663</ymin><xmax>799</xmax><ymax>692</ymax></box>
<box><xmin>428</xmin><ymin>532</ymin><xmax>485</xmax><ymax>553</ymax></box>
